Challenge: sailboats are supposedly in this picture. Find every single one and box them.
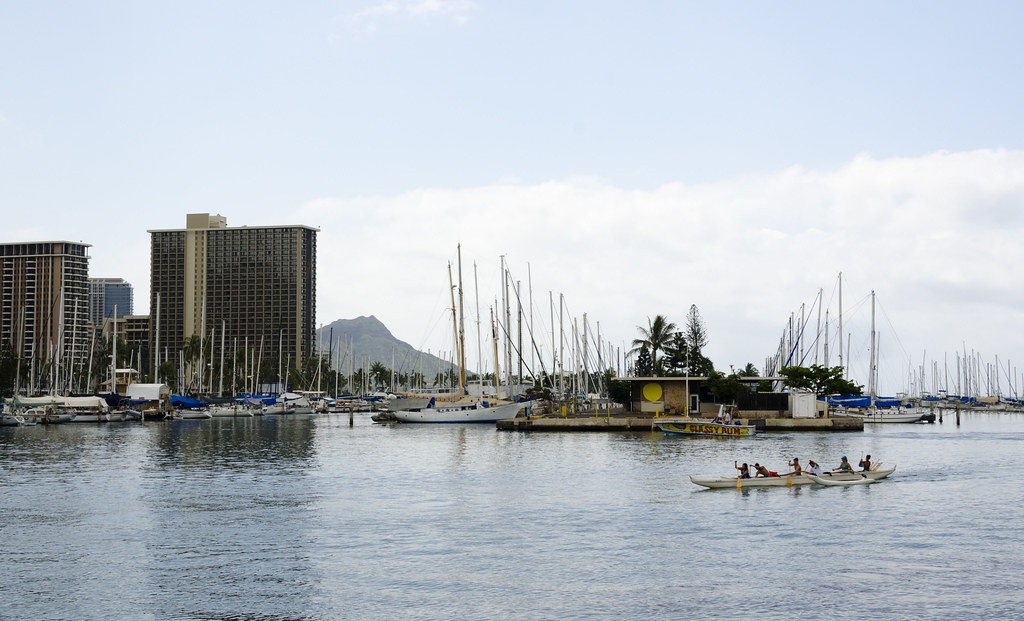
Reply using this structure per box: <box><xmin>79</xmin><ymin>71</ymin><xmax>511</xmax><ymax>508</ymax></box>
<box><xmin>0</xmin><ymin>241</ymin><xmax>1024</xmax><ymax>424</ymax></box>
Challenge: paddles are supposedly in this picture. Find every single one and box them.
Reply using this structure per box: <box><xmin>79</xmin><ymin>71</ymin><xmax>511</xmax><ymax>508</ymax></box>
<box><xmin>804</xmin><ymin>459</ymin><xmax>811</xmax><ymax>472</ymax></box>
<box><xmin>787</xmin><ymin>460</ymin><xmax>793</xmax><ymax>490</ymax></box>
<box><xmin>736</xmin><ymin>462</ymin><xmax>742</xmax><ymax>489</ymax></box>
<box><xmin>860</xmin><ymin>450</ymin><xmax>864</xmax><ymax>464</ymax></box>
<box><xmin>750</xmin><ymin>464</ymin><xmax>751</xmax><ymax>477</ymax></box>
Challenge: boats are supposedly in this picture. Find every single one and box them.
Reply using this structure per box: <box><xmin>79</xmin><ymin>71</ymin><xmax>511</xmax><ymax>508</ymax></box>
<box><xmin>652</xmin><ymin>419</ymin><xmax>757</xmax><ymax>437</ymax></box>
<box><xmin>688</xmin><ymin>464</ymin><xmax>899</xmax><ymax>488</ymax></box>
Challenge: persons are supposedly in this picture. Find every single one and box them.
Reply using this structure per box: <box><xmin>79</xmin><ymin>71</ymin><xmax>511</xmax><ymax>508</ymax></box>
<box><xmin>735</xmin><ymin>454</ymin><xmax>871</xmax><ymax>479</ymax></box>
<box><xmin>722</xmin><ymin>410</ymin><xmax>730</xmax><ymax>425</ymax></box>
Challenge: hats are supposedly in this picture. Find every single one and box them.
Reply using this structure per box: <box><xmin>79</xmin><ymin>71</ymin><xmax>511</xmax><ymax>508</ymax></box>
<box><xmin>841</xmin><ymin>456</ymin><xmax>846</xmax><ymax>460</ymax></box>
